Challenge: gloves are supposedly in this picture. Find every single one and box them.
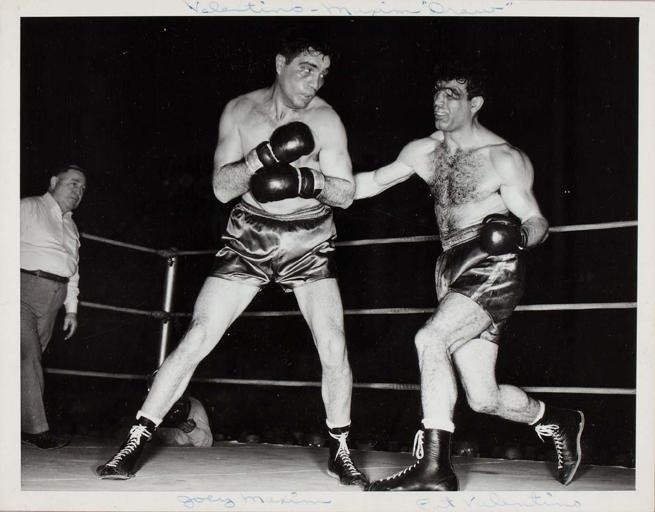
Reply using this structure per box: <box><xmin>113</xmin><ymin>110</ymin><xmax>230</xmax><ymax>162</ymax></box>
<box><xmin>250</xmin><ymin>164</ymin><xmax>325</xmax><ymax>203</ymax></box>
<box><xmin>245</xmin><ymin>121</ymin><xmax>315</xmax><ymax>174</ymax></box>
<box><xmin>479</xmin><ymin>214</ymin><xmax>529</xmax><ymax>256</ymax></box>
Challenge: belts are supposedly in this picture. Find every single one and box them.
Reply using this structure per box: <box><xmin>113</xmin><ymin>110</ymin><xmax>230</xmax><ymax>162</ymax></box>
<box><xmin>20</xmin><ymin>269</ymin><xmax>69</xmax><ymax>284</ymax></box>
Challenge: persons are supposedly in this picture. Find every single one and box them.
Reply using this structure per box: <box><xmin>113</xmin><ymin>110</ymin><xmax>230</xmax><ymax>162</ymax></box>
<box><xmin>20</xmin><ymin>164</ymin><xmax>86</xmax><ymax>450</ymax></box>
<box><xmin>216</xmin><ymin>416</ymin><xmax>635</xmax><ymax>465</ymax></box>
<box><xmin>95</xmin><ymin>39</ymin><xmax>372</xmax><ymax>488</ymax></box>
<box><xmin>342</xmin><ymin>65</ymin><xmax>584</xmax><ymax>491</ymax></box>
<box><xmin>144</xmin><ymin>372</ymin><xmax>213</xmax><ymax>448</ymax></box>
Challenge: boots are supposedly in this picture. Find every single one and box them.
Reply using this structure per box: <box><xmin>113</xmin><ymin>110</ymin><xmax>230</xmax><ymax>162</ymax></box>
<box><xmin>528</xmin><ymin>400</ymin><xmax>585</xmax><ymax>485</ymax></box>
<box><xmin>364</xmin><ymin>419</ymin><xmax>458</xmax><ymax>491</ymax></box>
<box><xmin>325</xmin><ymin>418</ymin><xmax>370</xmax><ymax>487</ymax></box>
<box><xmin>97</xmin><ymin>411</ymin><xmax>162</xmax><ymax>480</ymax></box>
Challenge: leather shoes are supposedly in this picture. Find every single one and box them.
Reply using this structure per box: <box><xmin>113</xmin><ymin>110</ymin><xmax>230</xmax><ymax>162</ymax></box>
<box><xmin>21</xmin><ymin>432</ymin><xmax>69</xmax><ymax>450</ymax></box>
